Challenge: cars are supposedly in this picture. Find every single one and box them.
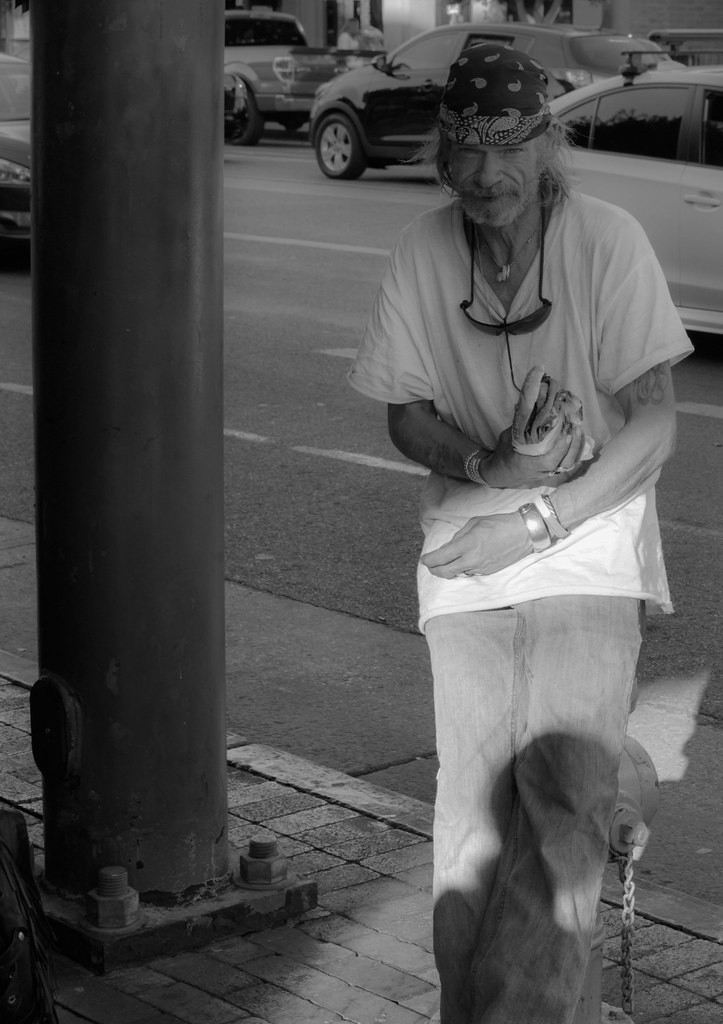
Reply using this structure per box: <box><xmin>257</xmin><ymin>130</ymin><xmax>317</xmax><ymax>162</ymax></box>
<box><xmin>542</xmin><ymin>63</ymin><xmax>723</xmax><ymax>342</ymax></box>
<box><xmin>305</xmin><ymin>18</ymin><xmax>671</xmax><ymax>184</ymax></box>
<box><xmin>1</xmin><ymin>51</ymin><xmax>33</xmax><ymax>268</ymax></box>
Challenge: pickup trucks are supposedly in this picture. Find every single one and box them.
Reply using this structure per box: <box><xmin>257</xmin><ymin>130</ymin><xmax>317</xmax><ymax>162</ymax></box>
<box><xmin>225</xmin><ymin>8</ymin><xmax>391</xmax><ymax>146</ymax></box>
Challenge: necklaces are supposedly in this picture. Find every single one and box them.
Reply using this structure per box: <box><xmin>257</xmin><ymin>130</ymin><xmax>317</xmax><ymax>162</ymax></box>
<box><xmin>473</xmin><ymin>211</ymin><xmax>541</xmax><ymax>283</ymax></box>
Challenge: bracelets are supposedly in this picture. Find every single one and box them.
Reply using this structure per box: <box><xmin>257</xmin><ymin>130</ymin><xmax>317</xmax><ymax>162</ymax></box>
<box><xmin>519</xmin><ymin>501</ymin><xmax>553</xmax><ymax>554</ymax></box>
<box><xmin>465</xmin><ymin>445</ymin><xmax>489</xmax><ymax>487</ymax></box>
<box><xmin>536</xmin><ymin>493</ymin><xmax>570</xmax><ymax>539</ymax></box>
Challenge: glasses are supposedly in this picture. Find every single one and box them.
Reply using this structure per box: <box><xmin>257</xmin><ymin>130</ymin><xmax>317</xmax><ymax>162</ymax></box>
<box><xmin>461</xmin><ymin>299</ymin><xmax>552</xmax><ymax>335</ymax></box>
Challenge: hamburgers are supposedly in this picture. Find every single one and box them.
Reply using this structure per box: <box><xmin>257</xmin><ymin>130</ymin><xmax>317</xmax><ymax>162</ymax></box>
<box><xmin>511</xmin><ymin>362</ymin><xmax>583</xmax><ymax>445</ymax></box>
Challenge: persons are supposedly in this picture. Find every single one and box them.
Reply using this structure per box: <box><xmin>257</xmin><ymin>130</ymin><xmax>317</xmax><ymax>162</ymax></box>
<box><xmin>343</xmin><ymin>42</ymin><xmax>699</xmax><ymax>1024</ymax></box>
